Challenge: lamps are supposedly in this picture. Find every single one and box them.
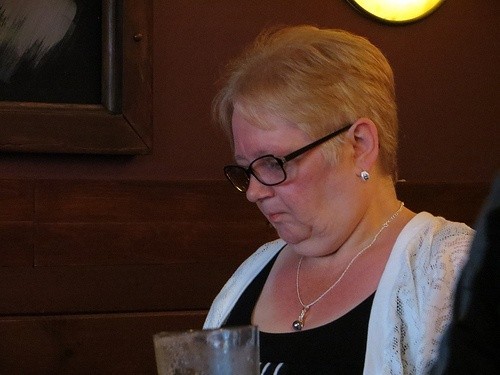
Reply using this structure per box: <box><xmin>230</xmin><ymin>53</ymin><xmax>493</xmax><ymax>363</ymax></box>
<box><xmin>345</xmin><ymin>0</ymin><xmax>444</xmax><ymax>24</ymax></box>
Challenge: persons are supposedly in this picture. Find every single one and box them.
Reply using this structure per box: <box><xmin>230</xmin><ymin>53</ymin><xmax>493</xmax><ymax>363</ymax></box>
<box><xmin>201</xmin><ymin>25</ymin><xmax>477</xmax><ymax>375</ymax></box>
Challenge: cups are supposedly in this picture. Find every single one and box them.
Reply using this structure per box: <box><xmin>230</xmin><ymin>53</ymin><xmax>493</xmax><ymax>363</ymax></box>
<box><xmin>152</xmin><ymin>324</ymin><xmax>260</xmax><ymax>375</ymax></box>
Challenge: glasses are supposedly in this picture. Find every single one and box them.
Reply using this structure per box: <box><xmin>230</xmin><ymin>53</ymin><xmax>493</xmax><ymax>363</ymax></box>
<box><xmin>223</xmin><ymin>124</ymin><xmax>354</xmax><ymax>193</ymax></box>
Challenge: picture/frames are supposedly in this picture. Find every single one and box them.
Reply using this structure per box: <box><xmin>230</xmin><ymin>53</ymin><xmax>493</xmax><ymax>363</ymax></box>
<box><xmin>0</xmin><ymin>0</ymin><xmax>153</xmax><ymax>155</ymax></box>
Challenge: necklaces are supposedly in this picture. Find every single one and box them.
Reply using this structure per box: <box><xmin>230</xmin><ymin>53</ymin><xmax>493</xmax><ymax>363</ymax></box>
<box><xmin>277</xmin><ymin>202</ymin><xmax>404</xmax><ymax>330</ymax></box>
